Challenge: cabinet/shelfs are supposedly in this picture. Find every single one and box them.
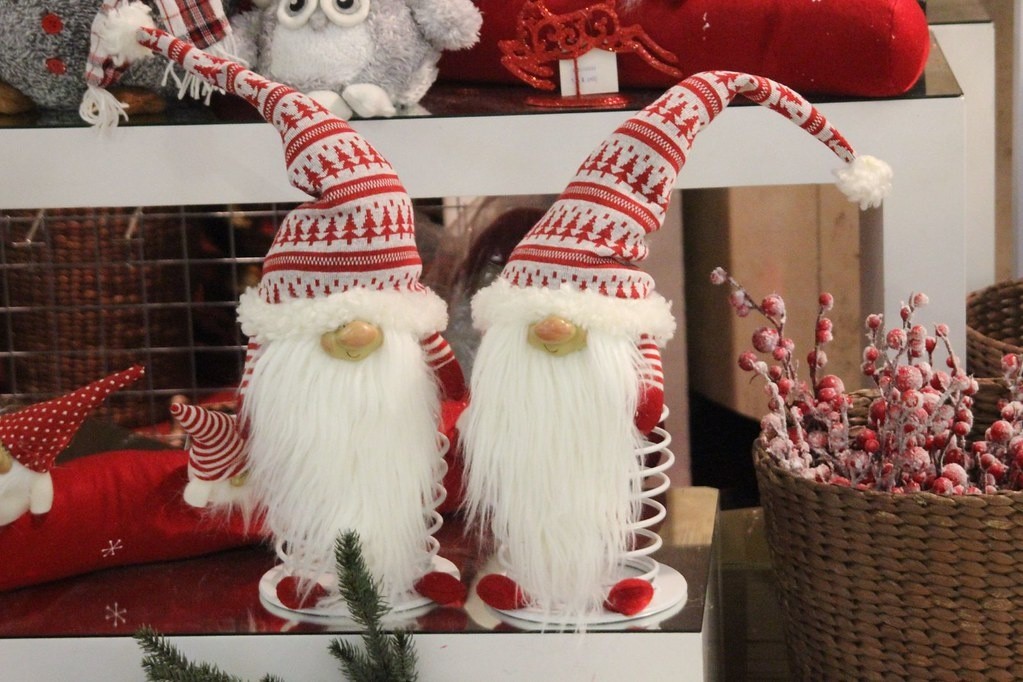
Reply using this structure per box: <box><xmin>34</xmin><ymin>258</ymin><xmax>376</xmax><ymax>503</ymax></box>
<box><xmin>0</xmin><ymin>26</ymin><xmax>969</xmax><ymax>682</ymax></box>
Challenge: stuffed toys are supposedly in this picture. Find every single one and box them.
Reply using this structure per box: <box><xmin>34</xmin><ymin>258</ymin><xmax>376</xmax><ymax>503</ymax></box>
<box><xmin>228</xmin><ymin>0</ymin><xmax>483</xmax><ymax>120</ymax></box>
<box><xmin>459</xmin><ymin>70</ymin><xmax>893</xmax><ymax>618</ymax></box>
<box><xmin>78</xmin><ymin>0</ymin><xmax>466</xmax><ymax>606</ymax></box>
<box><xmin>0</xmin><ymin>366</ymin><xmax>466</xmax><ymax>592</ymax></box>
